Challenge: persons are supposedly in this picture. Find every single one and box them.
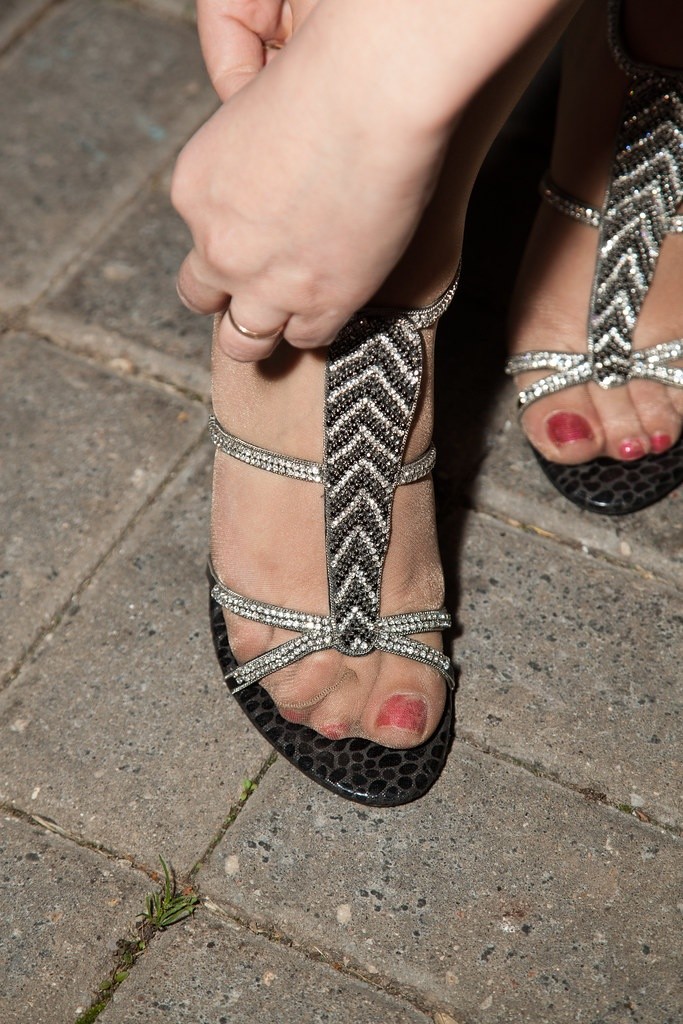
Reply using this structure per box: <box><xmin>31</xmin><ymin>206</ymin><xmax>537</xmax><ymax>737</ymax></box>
<box><xmin>167</xmin><ymin>0</ymin><xmax>683</xmax><ymax>809</ymax></box>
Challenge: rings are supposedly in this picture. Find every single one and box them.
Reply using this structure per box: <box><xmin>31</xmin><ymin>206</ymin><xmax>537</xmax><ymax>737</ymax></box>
<box><xmin>228</xmin><ymin>303</ymin><xmax>284</xmax><ymax>339</ymax></box>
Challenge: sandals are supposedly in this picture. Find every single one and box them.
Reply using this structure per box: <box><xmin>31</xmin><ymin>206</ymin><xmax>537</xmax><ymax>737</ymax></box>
<box><xmin>505</xmin><ymin>2</ymin><xmax>683</xmax><ymax>516</ymax></box>
<box><xmin>204</xmin><ymin>266</ymin><xmax>463</xmax><ymax>806</ymax></box>
